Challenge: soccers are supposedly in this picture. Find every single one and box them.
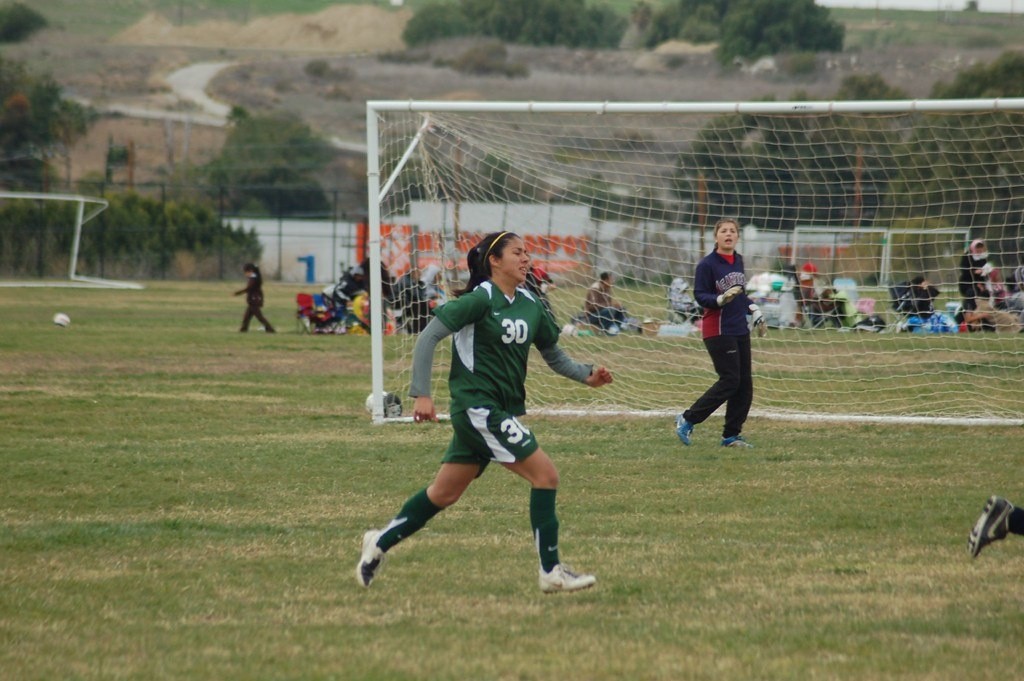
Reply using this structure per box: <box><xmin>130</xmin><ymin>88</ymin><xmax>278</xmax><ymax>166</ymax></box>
<box><xmin>365</xmin><ymin>390</ymin><xmax>389</xmax><ymax>414</ymax></box>
<box><xmin>54</xmin><ymin>313</ymin><xmax>70</xmax><ymax>327</ymax></box>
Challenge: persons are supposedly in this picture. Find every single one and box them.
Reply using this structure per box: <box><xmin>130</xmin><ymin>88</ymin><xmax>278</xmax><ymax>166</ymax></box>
<box><xmin>395</xmin><ymin>239</ymin><xmax>1024</xmax><ymax>338</ymax></box>
<box><xmin>233</xmin><ymin>264</ymin><xmax>277</xmax><ymax>333</ymax></box>
<box><xmin>970</xmin><ymin>495</ymin><xmax>1024</xmax><ymax>561</ymax></box>
<box><xmin>353</xmin><ymin>229</ymin><xmax>617</xmax><ymax>591</ymax></box>
<box><xmin>673</xmin><ymin>220</ymin><xmax>769</xmax><ymax>451</ymax></box>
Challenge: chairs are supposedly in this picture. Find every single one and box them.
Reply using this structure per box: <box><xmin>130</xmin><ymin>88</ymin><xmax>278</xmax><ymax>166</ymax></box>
<box><xmin>296</xmin><ymin>291</ymin><xmax>342</xmax><ymax>335</ymax></box>
<box><xmin>791</xmin><ymin>285</ymin><xmax>848</xmax><ymax>333</ymax></box>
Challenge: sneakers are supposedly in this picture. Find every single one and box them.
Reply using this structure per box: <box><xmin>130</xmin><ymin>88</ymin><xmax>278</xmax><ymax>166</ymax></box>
<box><xmin>538</xmin><ymin>564</ymin><xmax>596</xmax><ymax>595</ymax></box>
<box><xmin>720</xmin><ymin>435</ymin><xmax>753</xmax><ymax>450</ymax></box>
<box><xmin>967</xmin><ymin>494</ymin><xmax>1014</xmax><ymax>559</ymax></box>
<box><xmin>675</xmin><ymin>413</ymin><xmax>694</xmax><ymax>446</ymax></box>
<box><xmin>356</xmin><ymin>529</ymin><xmax>387</xmax><ymax>589</ymax></box>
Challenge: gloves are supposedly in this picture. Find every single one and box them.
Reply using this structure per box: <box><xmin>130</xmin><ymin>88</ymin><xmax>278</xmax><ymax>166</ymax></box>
<box><xmin>748</xmin><ymin>302</ymin><xmax>767</xmax><ymax>337</ymax></box>
<box><xmin>717</xmin><ymin>286</ymin><xmax>743</xmax><ymax>307</ymax></box>
